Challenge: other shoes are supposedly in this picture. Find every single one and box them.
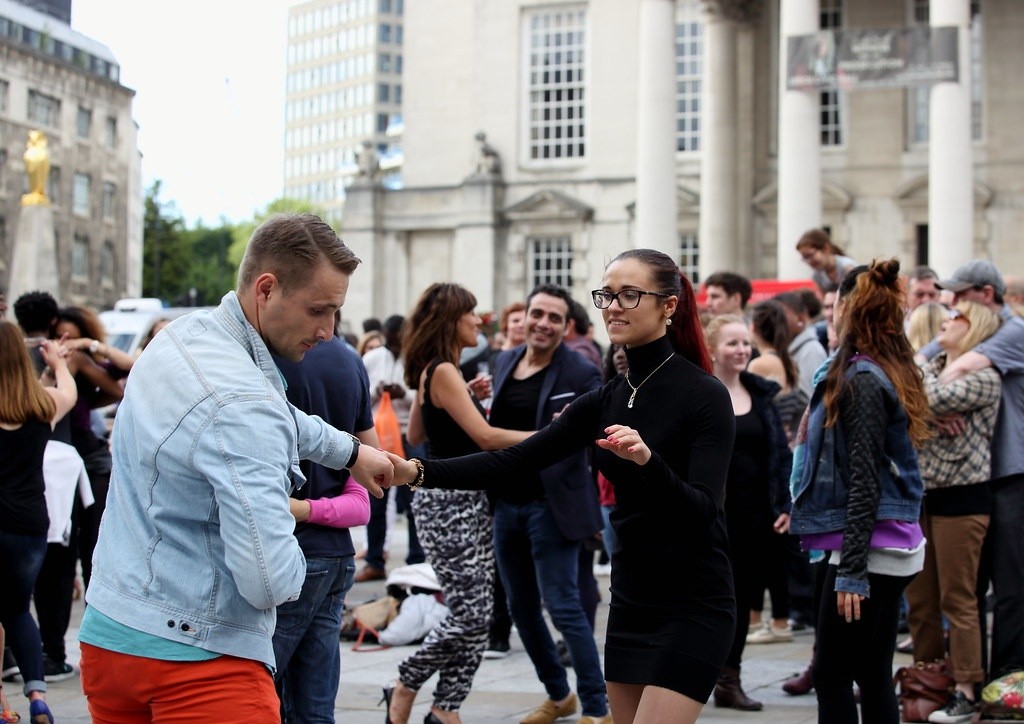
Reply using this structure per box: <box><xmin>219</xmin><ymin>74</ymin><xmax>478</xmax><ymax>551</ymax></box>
<box><xmin>576</xmin><ymin>713</ymin><xmax>613</xmax><ymax>724</ymax></box>
<box><xmin>520</xmin><ymin>694</ymin><xmax>576</xmax><ymax>724</ymax></box>
<box><xmin>782</xmin><ymin>652</ymin><xmax>816</xmax><ymax>694</ymax></box>
<box><xmin>354</xmin><ymin>564</ymin><xmax>385</xmax><ymax>582</ymax></box>
<box><xmin>0</xmin><ymin>709</ymin><xmax>20</xmax><ymax>723</ymax></box>
<box><xmin>44</xmin><ymin>662</ymin><xmax>75</xmax><ymax>681</ymax></box>
<box><xmin>2</xmin><ymin>648</ymin><xmax>20</xmax><ymax>680</ymax></box>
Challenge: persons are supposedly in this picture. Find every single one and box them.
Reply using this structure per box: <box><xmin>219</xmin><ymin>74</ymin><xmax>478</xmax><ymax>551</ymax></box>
<box><xmin>697</xmin><ymin>224</ymin><xmax>1024</xmax><ymax>724</ymax></box>
<box><xmin>0</xmin><ymin>292</ymin><xmax>173</xmax><ymax>724</ymax></box>
<box><xmin>374</xmin><ymin>249</ymin><xmax>738</xmax><ymax>724</ymax></box>
<box><xmin>78</xmin><ymin>211</ymin><xmax>395</xmax><ymax>724</ymax></box>
<box><xmin>267</xmin><ymin>283</ymin><xmax>628</xmax><ymax>724</ymax></box>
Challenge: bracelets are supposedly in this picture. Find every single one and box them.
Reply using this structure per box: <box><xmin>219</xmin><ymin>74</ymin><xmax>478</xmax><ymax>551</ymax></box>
<box><xmin>45</xmin><ymin>368</ymin><xmax>55</xmax><ymax>380</ymax></box>
<box><xmin>345</xmin><ymin>434</ymin><xmax>361</xmax><ymax>470</ymax></box>
<box><xmin>405</xmin><ymin>457</ymin><xmax>424</xmax><ymax>493</ymax></box>
<box><xmin>104</xmin><ymin>344</ymin><xmax>109</xmax><ymax>357</ymax></box>
<box><xmin>90</xmin><ymin>341</ymin><xmax>99</xmax><ymax>353</ymax></box>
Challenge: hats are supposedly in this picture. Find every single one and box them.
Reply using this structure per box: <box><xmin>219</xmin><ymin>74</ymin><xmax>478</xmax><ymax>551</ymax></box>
<box><xmin>933</xmin><ymin>260</ymin><xmax>1007</xmax><ymax>296</ymax></box>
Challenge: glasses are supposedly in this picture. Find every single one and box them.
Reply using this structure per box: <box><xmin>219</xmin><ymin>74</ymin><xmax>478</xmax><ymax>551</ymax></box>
<box><xmin>592</xmin><ymin>289</ymin><xmax>671</xmax><ymax>310</ymax></box>
<box><xmin>801</xmin><ymin>249</ymin><xmax>818</xmax><ymax>260</ymax></box>
<box><xmin>947</xmin><ymin>309</ymin><xmax>964</xmax><ymax>322</ymax></box>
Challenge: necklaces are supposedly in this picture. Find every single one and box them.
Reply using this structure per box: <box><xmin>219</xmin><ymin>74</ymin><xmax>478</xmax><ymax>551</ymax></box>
<box><xmin>626</xmin><ymin>352</ymin><xmax>676</xmax><ymax>409</ymax></box>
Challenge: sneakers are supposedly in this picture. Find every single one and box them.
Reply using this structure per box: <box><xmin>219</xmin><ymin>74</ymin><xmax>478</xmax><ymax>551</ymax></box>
<box><xmin>746</xmin><ymin>617</ymin><xmax>793</xmax><ymax>644</ymax></box>
<box><xmin>928</xmin><ymin>691</ymin><xmax>983</xmax><ymax>724</ymax></box>
<box><xmin>484</xmin><ymin>638</ymin><xmax>513</xmax><ymax>657</ymax></box>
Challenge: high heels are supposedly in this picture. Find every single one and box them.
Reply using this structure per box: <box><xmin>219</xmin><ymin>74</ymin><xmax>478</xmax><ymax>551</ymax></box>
<box><xmin>29</xmin><ymin>700</ymin><xmax>54</xmax><ymax>724</ymax></box>
<box><xmin>714</xmin><ymin>669</ymin><xmax>762</xmax><ymax>711</ymax></box>
<box><xmin>378</xmin><ymin>688</ymin><xmax>409</xmax><ymax>724</ymax></box>
<box><xmin>424</xmin><ymin>711</ymin><xmax>447</xmax><ymax>724</ymax></box>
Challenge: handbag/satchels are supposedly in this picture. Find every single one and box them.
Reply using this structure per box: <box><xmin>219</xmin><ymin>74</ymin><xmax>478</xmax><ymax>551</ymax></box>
<box><xmin>893</xmin><ymin>656</ymin><xmax>954</xmax><ymax>721</ymax></box>
<box><xmin>374</xmin><ymin>392</ymin><xmax>407</xmax><ymax>462</ymax></box>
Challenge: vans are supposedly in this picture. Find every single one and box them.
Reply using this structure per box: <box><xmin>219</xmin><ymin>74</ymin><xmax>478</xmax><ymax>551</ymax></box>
<box><xmin>99</xmin><ymin>298</ymin><xmax>219</xmax><ymax>350</ymax></box>
